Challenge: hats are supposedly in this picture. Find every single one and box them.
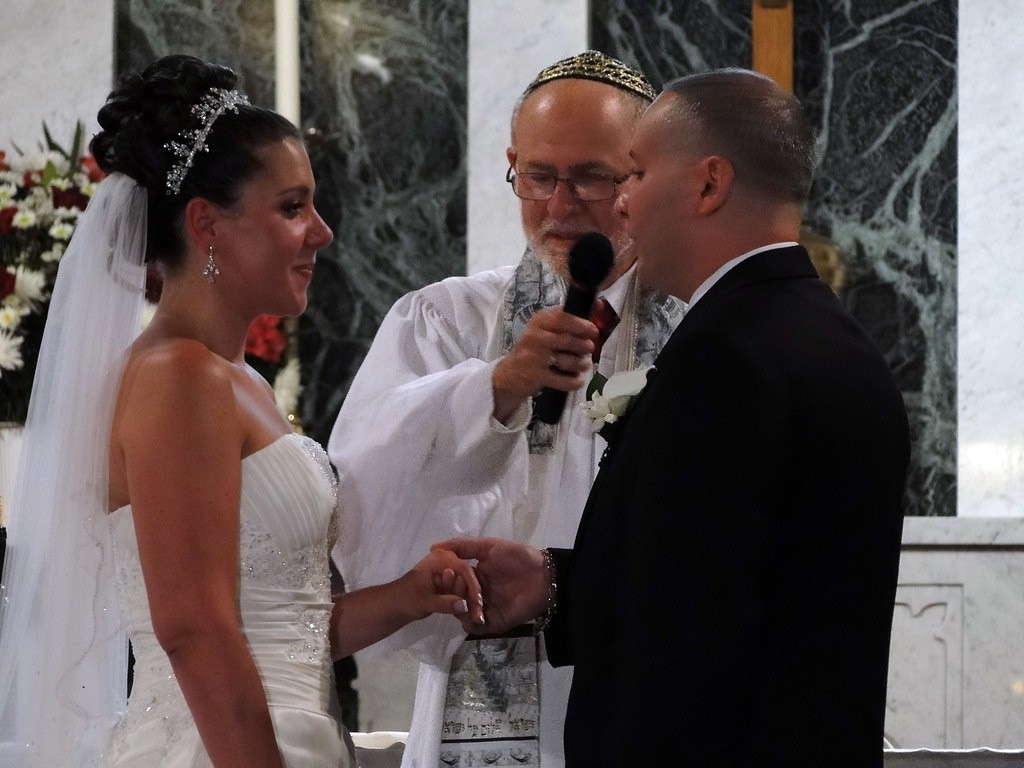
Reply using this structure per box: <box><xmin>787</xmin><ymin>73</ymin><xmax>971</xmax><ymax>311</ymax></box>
<box><xmin>521</xmin><ymin>49</ymin><xmax>658</xmax><ymax>103</ymax></box>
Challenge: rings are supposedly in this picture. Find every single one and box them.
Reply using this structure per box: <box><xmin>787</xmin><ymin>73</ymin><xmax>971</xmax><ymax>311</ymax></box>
<box><xmin>545</xmin><ymin>352</ymin><xmax>560</xmax><ymax>368</ymax></box>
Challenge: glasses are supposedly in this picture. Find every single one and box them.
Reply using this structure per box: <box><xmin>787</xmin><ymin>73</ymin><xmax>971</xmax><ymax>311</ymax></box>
<box><xmin>504</xmin><ymin>160</ymin><xmax>631</xmax><ymax>204</ymax></box>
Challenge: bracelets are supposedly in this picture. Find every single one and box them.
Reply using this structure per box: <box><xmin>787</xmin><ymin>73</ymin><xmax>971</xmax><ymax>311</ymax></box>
<box><xmin>534</xmin><ymin>547</ymin><xmax>558</xmax><ymax>632</ymax></box>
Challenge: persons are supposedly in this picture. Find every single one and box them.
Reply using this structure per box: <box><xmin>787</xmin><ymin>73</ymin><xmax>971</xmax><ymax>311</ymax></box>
<box><xmin>327</xmin><ymin>49</ymin><xmax>907</xmax><ymax>768</ymax></box>
<box><xmin>0</xmin><ymin>47</ymin><xmax>484</xmax><ymax>768</ymax></box>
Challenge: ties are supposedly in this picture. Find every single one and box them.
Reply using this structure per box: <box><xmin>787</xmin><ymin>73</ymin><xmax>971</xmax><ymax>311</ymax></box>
<box><xmin>584</xmin><ymin>297</ymin><xmax>620</xmax><ymax>363</ymax></box>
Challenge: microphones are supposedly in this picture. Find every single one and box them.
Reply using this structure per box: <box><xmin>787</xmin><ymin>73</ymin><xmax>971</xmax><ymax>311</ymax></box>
<box><xmin>535</xmin><ymin>231</ymin><xmax>614</xmax><ymax>424</ymax></box>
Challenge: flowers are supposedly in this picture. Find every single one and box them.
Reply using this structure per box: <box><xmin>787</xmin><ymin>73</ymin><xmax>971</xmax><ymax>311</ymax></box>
<box><xmin>0</xmin><ymin>118</ymin><xmax>305</xmax><ymax>422</ymax></box>
<box><xmin>579</xmin><ymin>363</ymin><xmax>656</xmax><ymax>434</ymax></box>
